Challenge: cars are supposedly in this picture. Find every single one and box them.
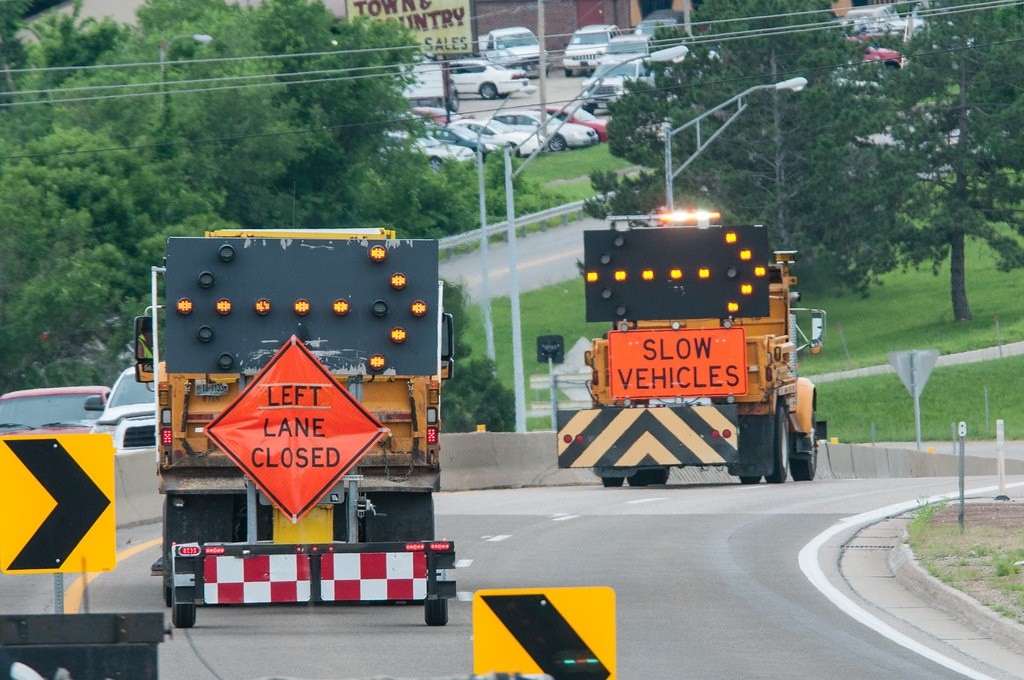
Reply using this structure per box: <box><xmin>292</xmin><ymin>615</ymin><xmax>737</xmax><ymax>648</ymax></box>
<box><xmin>0</xmin><ymin>385</ymin><xmax>110</xmax><ymax>434</ymax></box>
<box><xmin>837</xmin><ymin>2</ymin><xmax>925</xmax><ymax>71</ymax></box>
<box><xmin>378</xmin><ymin>9</ymin><xmax>686</xmax><ymax>175</ymax></box>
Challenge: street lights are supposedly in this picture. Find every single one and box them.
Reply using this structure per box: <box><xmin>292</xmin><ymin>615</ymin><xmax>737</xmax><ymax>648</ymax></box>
<box><xmin>158</xmin><ymin>30</ymin><xmax>216</xmax><ymax>80</ymax></box>
<box><xmin>662</xmin><ymin>75</ymin><xmax>808</xmax><ymax>212</ymax></box>
<box><xmin>503</xmin><ymin>42</ymin><xmax>691</xmax><ymax>433</ymax></box>
<box><xmin>474</xmin><ymin>84</ymin><xmax>539</xmax><ymax>375</ymax></box>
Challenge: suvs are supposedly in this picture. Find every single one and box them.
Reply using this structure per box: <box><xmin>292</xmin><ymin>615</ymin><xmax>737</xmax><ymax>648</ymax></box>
<box><xmin>84</xmin><ymin>366</ymin><xmax>157</xmax><ymax>450</ymax></box>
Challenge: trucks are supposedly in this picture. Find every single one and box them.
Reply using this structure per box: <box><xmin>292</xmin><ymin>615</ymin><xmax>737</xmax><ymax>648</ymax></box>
<box><xmin>133</xmin><ymin>225</ymin><xmax>456</xmax><ymax>629</ymax></box>
<box><xmin>558</xmin><ymin>211</ymin><xmax>826</xmax><ymax>488</ymax></box>
